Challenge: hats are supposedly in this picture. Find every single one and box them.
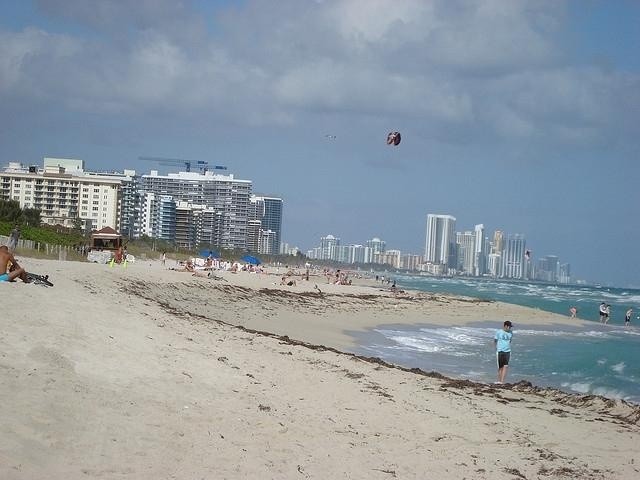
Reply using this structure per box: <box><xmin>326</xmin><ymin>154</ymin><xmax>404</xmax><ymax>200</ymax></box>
<box><xmin>504</xmin><ymin>321</ymin><xmax>513</xmax><ymax>327</ymax></box>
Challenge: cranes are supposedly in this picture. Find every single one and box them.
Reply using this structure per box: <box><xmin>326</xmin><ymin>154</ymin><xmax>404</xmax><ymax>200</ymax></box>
<box><xmin>135</xmin><ymin>154</ymin><xmax>209</xmax><ymax>173</ymax></box>
<box><xmin>157</xmin><ymin>161</ymin><xmax>228</xmax><ymax>174</ymax></box>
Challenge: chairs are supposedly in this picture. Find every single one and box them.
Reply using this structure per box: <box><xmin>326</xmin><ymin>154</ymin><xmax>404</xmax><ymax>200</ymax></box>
<box><xmin>191</xmin><ymin>257</ymin><xmax>241</xmax><ymax>271</ymax></box>
<box><xmin>86</xmin><ymin>249</ymin><xmax>135</xmax><ymax>264</ymax></box>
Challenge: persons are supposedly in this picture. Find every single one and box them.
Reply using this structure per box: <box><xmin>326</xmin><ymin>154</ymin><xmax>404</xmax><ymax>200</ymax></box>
<box><xmin>8</xmin><ymin>224</ymin><xmax>21</xmax><ymax>255</ymax></box>
<box><xmin>115</xmin><ymin>247</ymin><xmax>122</xmax><ymax>265</ymax></box>
<box><xmin>0</xmin><ymin>245</ymin><xmax>31</xmax><ymax>283</ymax></box>
<box><xmin>159</xmin><ymin>251</ymin><xmax>397</xmax><ymax>288</ymax></box>
<box><xmin>123</xmin><ymin>246</ymin><xmax>127</xmax><ymax>261</ymax></box>
<box><xmin>494</xmin><ymin>320</ymin><xmax>514</xmax><ymax>385</ymax></box>
<box><xmin>570</xmin><ymin>302</ymin><xmax>633</xmax><ymax>328</ymax></box>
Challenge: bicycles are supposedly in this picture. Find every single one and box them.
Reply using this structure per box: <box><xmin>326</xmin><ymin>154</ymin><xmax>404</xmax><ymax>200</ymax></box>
<box><xmin>7</xmin><ymin>261</ymin><xmax>55</xmax><ymax>288</ymax></box>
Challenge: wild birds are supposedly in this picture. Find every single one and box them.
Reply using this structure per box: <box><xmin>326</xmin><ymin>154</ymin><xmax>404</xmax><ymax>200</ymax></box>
<box><xmin>387</xmin><ymin>131</ymin><xmax>401</xmax><ymax>146</ymax></box>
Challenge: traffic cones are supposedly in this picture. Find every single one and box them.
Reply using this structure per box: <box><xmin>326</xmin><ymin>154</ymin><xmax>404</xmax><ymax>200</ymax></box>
<box><xmin>122</xmin><ymin>258</ymin><xmax>129</xmax><ymax>270</ymax></box>
<box><xmin>110</xmin><ymin>258</ymin><xmax>116</xmax><ymax>268</ymax></box>
